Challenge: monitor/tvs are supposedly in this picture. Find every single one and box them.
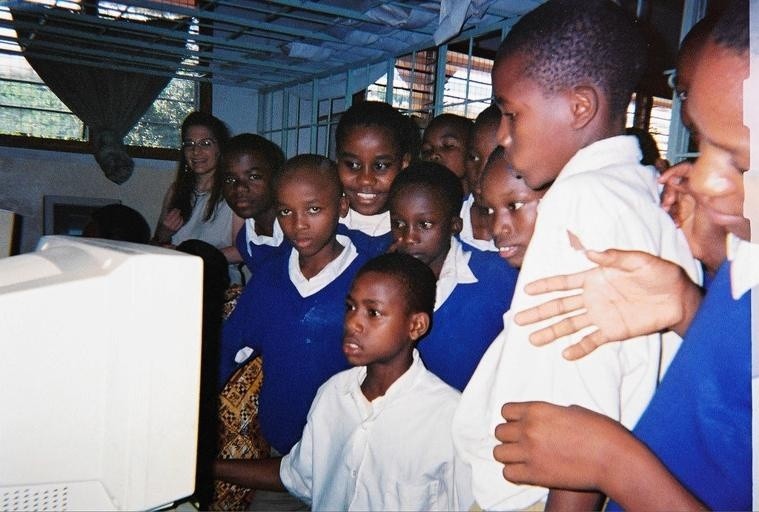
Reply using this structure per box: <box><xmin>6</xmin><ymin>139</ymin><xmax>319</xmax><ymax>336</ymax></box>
<box><xmin>44</xmin><ymin>194</ymin><xmax>122</xmax><ymax>236</ymax></box>
<box><xmin>0</xmin><ymin>235</ymin><xmax>204</xmax><ymax>512</ymax></box>
<box><xmin>0</xmin><ymin>208</ymin><xmax>16</xmax><ymax>260</ymax></box>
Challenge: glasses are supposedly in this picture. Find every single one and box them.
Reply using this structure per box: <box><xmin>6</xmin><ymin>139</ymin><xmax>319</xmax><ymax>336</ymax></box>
<box><xmin>183</xmin><ymin>140</ymin><xmax>217</xmax><ymax>150</ymax></box>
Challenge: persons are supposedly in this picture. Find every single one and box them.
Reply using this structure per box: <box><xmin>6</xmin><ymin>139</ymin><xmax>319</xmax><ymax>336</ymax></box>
<box><xmin>79</xmin><ymin>0</ymin><xmax>750</xmax><ymax>511</ymax></box>
